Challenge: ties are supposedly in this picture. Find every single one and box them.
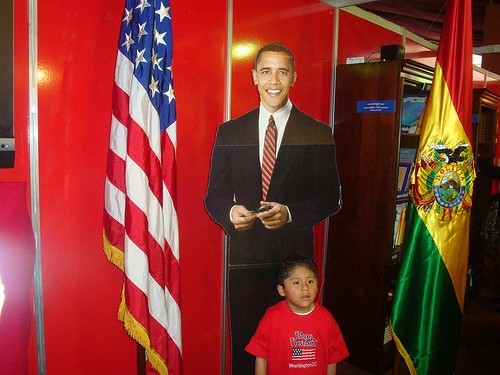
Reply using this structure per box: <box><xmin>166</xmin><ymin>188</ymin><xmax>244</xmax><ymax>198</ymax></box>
<box><xmin>261</xmin><ymin>115</ymin><xmax>278</xmax><ymax>201</ymax></box>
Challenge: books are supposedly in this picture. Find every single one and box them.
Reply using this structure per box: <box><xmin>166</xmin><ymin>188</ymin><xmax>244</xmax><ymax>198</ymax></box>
<box><xmin>393</xmin><ymin>95</ymin><xmax>428</xmax><ymax>247</ymax></box>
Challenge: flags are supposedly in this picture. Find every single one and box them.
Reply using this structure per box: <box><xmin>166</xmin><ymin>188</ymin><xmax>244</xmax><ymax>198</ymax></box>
<box><xmin>103</xmin><ymin>0</ymin><xmax>185</xmax><ymax>375</ymax></box>
<box><xmin>377</xmin><ymin>0</ymin><xmax>475</xmax><ymax>375</ymax></box>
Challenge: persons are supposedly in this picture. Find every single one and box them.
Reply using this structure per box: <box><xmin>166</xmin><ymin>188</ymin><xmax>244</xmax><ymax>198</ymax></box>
<box><xmin>243</xmin><ymin>254</ymin><xmax>349</xmax><ymax>375</ymax></box>
<box><xmin>465</xmin><ymin>143</ymin><xmax>493</xmax><ymax>272</ymax></box>
<box><xmin>203</xmin><ymin>42</ymin><xmax>342</xmax><ymax>375</ymax></box>
